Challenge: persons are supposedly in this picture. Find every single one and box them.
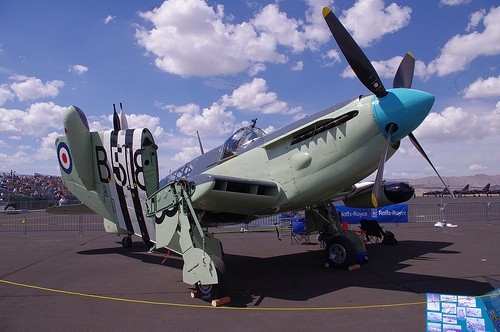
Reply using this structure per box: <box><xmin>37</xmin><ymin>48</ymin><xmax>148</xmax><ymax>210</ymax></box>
<box><xmin>0</xmin><ymin>170</ymin><xmax>77</xmax><ymax>200</ymax></box>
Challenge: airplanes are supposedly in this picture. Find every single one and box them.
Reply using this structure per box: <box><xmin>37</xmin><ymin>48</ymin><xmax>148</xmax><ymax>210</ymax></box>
<box><xmin>423</xmin><ymin>183</ymin><xmax>500</xmax><ymax>197</ymax></box>
<box><xmin>46</xmin><ymin>7</ymin><xmax>457</xmax><ymax>302</ymax></box>
